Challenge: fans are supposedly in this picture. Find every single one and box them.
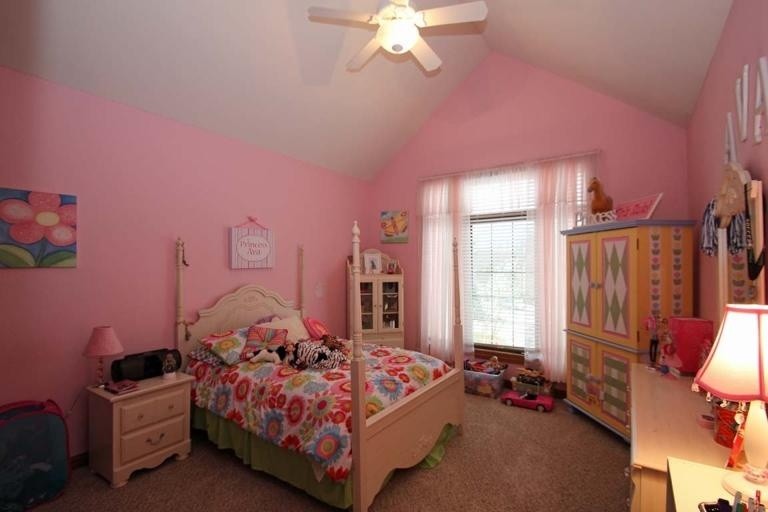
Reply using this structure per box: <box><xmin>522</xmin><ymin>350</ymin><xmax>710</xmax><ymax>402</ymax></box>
<box><xmin>301</xmin><ymin>0</ymin><xmax>486</xmax><ymax>75</ymax></box>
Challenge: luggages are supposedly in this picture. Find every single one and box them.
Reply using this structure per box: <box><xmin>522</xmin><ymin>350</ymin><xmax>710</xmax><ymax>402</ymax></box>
<box><xmin>1</xmin><ymin>398</ymin><xmax>73</xmax><ymax>512</ymax></box>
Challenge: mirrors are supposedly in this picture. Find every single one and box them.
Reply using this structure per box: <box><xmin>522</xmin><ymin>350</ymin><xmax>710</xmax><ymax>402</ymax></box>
<box><xmin>713</xmin><ymin>162</ymin><xmax>766</xmax><ymax>345</ymax></box>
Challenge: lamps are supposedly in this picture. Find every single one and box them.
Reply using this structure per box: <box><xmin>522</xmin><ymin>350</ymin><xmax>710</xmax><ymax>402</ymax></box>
<box><xmin>378</xmin><ymin>12</ymin><xmax>420</xmax><ymax>55</ymax></box>
<box><xmin>78</xmin><ymin>322</ymin><xmax>122</xmax><ymax>378</ymax></box>
<box><xmin>690</xmin><ymin>300</ymin><xmax>768</xmax><ymax>485</ymax></box>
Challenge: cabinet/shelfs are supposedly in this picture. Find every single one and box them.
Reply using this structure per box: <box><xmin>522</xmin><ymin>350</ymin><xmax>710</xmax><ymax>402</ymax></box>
<box><xmin>559</xmin><ymin>216</ymin><xmax>703</xmax><ymax>445</ymax></box>
<box><xmin>615</xmin><ymin>360</ymin><xmax>768</xmax><ymax>512</ymax></box>
<box><xmin>343</xmin><ymin>249</ymin><xmax>406</xmax><ymax>348</ymax></box>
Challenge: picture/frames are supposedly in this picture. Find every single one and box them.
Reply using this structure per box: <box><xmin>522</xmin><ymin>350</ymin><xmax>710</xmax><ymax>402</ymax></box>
<box><xmin>386</xmin><ymin>261</ymin><xmax>397</xmax><ymax>273</ymax></box>
<box><xmin>610</xmin><ymin>191</ymin><xmax>665</xmax><ymax>221</ymax></box>
<box><xmin>363</xmin><ymin>252</ymin><xmax>384</xmax><ymax>275</ymax></box>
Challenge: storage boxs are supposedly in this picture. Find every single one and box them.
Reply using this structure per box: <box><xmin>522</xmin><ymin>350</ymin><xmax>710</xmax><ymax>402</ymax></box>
<box><xmin>511</xmin><ymin>372</ymin><xmax>554</xmax><ymax>395</ymax></box>
<box><xmin>460</xmin><ymin>363</ymin><xmax>507</xmax><ymax>399</ymax></box>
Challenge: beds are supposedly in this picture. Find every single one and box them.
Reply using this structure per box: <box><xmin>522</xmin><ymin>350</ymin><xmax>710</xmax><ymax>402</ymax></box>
<box><xmin>175</xmin><ymin>220</ymin><xmax>472</xmax><ymax>512</ymax></box>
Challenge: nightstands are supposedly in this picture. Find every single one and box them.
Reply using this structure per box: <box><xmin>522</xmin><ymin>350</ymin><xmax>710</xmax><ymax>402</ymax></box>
<box><xmin>87</xmin><ymin>372</ymin><xmax>199</xmax><ymax>489</ymax></box>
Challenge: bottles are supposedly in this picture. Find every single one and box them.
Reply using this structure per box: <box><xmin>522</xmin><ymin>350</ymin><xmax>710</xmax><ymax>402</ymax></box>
<box><xmin>164</xmin><ymin>354</ymin><xmax>176</xmax><ymax>379</ymax></box>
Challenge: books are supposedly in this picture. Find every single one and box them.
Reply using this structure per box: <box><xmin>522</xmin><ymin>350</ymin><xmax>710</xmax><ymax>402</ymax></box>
<box><xmin>104</xmin><ymin>379</ymin><xmax>141</xmax><ymax>396</ymax></box>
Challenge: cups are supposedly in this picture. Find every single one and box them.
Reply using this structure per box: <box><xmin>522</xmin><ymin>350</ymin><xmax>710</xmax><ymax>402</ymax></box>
<box><xmin>387</xmin><ymin>263</ymin><xmax>395</xmax><ymax>274</ymax></box>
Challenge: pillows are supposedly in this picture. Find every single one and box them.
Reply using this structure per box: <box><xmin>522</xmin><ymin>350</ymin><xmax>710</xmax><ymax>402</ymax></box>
<box><xmin>300</xmin><ymin>316</ymin><xmax>332</xmax><ymax>339</ymax></box>
<box><xmin>186</xmin><ymin>344</ymin><xmax>221</xmax><ymax>369</ymax></box>
<box><xmin>240</xmin><ymin>324</ymin><xmax>288</xmax><ymax>362</ymax></box>
<box><xmin>256</xmin><ymin>315</ymin><xmax>306</xmax><ymax>341</ymax></box>
<box><xmin>201</xmin><ymin>324</ymin><xmax>251</xmax><ymax>362</ymax></box>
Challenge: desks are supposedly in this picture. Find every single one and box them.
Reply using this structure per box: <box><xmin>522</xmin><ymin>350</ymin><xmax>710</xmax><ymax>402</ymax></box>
<box><xmin>661</xmin><ymin>453</ymin><xmax>768</xmax><ymax>512</ymax></box>
